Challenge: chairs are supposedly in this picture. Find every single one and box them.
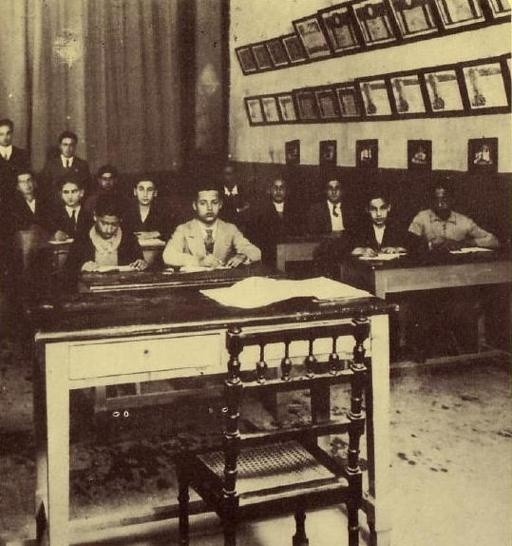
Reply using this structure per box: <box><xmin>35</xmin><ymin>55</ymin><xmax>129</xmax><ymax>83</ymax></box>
<box><xmin>173</xmin><ymin>311</ymin><xmax>372</xmax><ymax>546</ymax></box>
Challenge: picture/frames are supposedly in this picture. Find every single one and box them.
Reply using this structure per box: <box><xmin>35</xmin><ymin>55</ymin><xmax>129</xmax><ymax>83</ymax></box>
<box><xmin>348</xmin><ymin>0</ymin><xmax>400</xmax><ymax>49</ymax></box>
<box><xmin>421</xmin><ymin>64</ymin><xmax>468</xmax><ymax>119</ymax></box>
<box><xmin>467</xmin><ymin>138</ymin><xmax>500</xmax><ymax>173</ymax></box>
<box><xmin>318</xmin><ymin>2</ymin><xmax>363</xmax><ymax>58</ymax></box>
<box><xmin>264</xmin><ymin>37</ymin><xmax>290</xmax><ymax>69</ymax></box>
<box><xmin>333</xmin><ymin>82</ymin><xmax>362</xmax><ymax>122</ymax></box>
<box><xmin>433</xmin><ymin>0</ymin><xmax>491</xmax><ymax>33</ymax></box>
<box><xmin>276</xmin><ymin>92</ymin><xmax>298</xmax><ymax>124</ymax></box>
<box><xmin>250</xmin><ymin>42</ymin><xmax>273</xmax><ymax>71</ymax></box>
<box><xmin>292</xmin><ymin>13</ymin><xmax>333</xmax><ymax>61</ymax></box>
<box><xmin>320</xmin><ymin>141</ymin><xmax>335</xmax><ymax>164</ymax></box>
<box><xmin>280</xmin><ymin>32</ymin><xmax>308</xmax><ymax>65</ymax></box>
<box><xmin>387</xmin><ymin>0</ymin><xmax>443</xmax><ymax>42</ymax></box>
<box><xmin>260</xmin><ymin>94</ymin><xmax>281</xmax><ymax>125</ymax></box>
<box><xmin>485</xmin><ymin>0</ymin><xmax>512</xmax><ymax>25</ymax></box>
<box><xmin>502</xmin><ymin>52</ymin><xmax>512</xmax><ymax>88</ymax></box>
<box><xmin>235</xmin><ymin>46</ymin><xmax>257</xmax><ymax>76</ymax></box>
<box><xmin>407</xmin><ymin>140</ymin><xmax>432</xmax><ymax>173</ymax></box>
<box><xmin>243</xmin><ymin>96</ymin><xmax>265</xmax><ymax>127</ymax></box>
<box><xmin>457</xmin><ymin>56</ymin><xmax>510</xmax><ymax>114</ymax></box>
<box><xmin>387</xmin><ymin>69</ymin><xmax>430</xmax><ymax>120</ymax></box>
<box><xmin>313</xmin><ymin>84</ymin><xmax>341</xmax><ymax>123</ymax></box>
<box><xmin>293</xmin><ymin>87</ymin><xmax>320</xmax><ymax>124</ymax></box>
<box><xmin>355</xmin><ymin>74</ymin><xmax>395</xmax><ymax>121</ymax></box>
<box><xmin>357</xmin><ymin>140</ymin><xmax>376</xmax><ymax>169</ymax></box>
<box><xmin>287</xmin><ymin>140</ymin><xmax>301</xmax><ymax>167</ymax></box>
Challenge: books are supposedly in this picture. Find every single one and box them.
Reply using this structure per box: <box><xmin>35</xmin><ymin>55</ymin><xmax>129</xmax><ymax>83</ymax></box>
<box><xmin>93</xmin><ymin>264</ymin><xmax>138</xmax><ymax>274</ymax></box>
<box><xmin>47</xmin><ymin>239</ymin><xmax>75</xmax><ymax>246</ymax></box>
<box><xmin>138</xmin><ymin>238</ymin><xmax>166</xmax><ymax>247</ymax></box>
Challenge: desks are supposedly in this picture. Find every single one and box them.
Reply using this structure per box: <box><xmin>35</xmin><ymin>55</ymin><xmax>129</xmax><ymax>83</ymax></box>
<box><xmin>13</xmin><ymin>230</ymin><xmax>163</xmax><ymax>267</ymax></box>
<box><xmin>272</xmin><ymin>234</ymin><xmax>341</xmax><ymax>272</ymax></box>
<box><xmin>341</xmin><ymin>246</ymin><xmax>512</xmax><ymax>368</ymax></box>
<box><xmin>79</xmin><ymin>258</ymin><xmax>280</xmax><ymax>413</ymax></box>
<box><xmin>28</xmin><ymin>290</ymin><xmax>399</xmax><ymax>546</ymax></box>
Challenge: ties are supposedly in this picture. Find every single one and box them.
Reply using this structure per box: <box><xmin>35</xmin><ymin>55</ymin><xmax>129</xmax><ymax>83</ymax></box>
<box><xmin>2</xmin><ymin>153</ymin><xmax>9</xmax><ymax>162</ymax></box>
<box><xmin>65</xmin><ymin>160</ymin><xmax>69</xmax><ymax>171</ymax></box>
<box><xmin>70</xmin><ymin>210</ymin><xmax>76</xmax><ymax>229</ymax></box>
<box><xmin>332</xmin><ymin>205</ymin><xmax>338</xmax><ymax>217</ymax></box>
<box><xmin>203</xmin><ymin>229</ymin><xmax>215</xmax><ymax>256</ymax></box>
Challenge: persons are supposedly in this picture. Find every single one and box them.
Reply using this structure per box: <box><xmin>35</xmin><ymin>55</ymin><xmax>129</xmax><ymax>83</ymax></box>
<box><xmin>0</xmin><ymin>118</ymin><xmax>31</xmax><ymax>172</ymax></box>
<box><xmin>406</xmin><ymin>181</ymin><xmax>500</xmax><ymax>355</ymax></box>
<box><xmin>41</xmin><ymin>175</ymin><xmax>90</xmax><ymax>242</ymax></box>
<box><xmin>214</xmin><ymin>161</ymin><xmax>253</xmax><ymax>217</ymax></box>
<box><xmin>43</xmin><ymin>130</ymin><xmax>90</xmax><ymax>183</ymax></box>
<box><xmin>5</xmin><ymin>168</ymin><xmax>50</xmax><ymax>262</ymax></box>
<box><xmin>64</xmin><ymin>190</ymin><xmax>148</xmax><ymax>275</ymax></box>
<box><xmin>121</xmin><ymin>172</ymin><xmax>178</xmax><ymax>241</ymax></box>
<box><xmin>83</xmin><ymin>162</ymin><xmax>130</xmax><ymax>225</ymax></box>
<box><xmin>160</xmin><ymin>181</ymin><xmax>263</xmax><ymax>272</ymax></box>
<box><xmin>243</xmin><ymin>174</ymin><xmax>310</xmax><ymax>235</ymax></box>
<box><xmin>338</xmin><ymin>188</ymin><xmax>411</xmax><ymax>362</ymax></box>
<box><xmin>311</xmin><ymin>174</ymin><xmax>356</xmax><ymax>234</ymax></box>
